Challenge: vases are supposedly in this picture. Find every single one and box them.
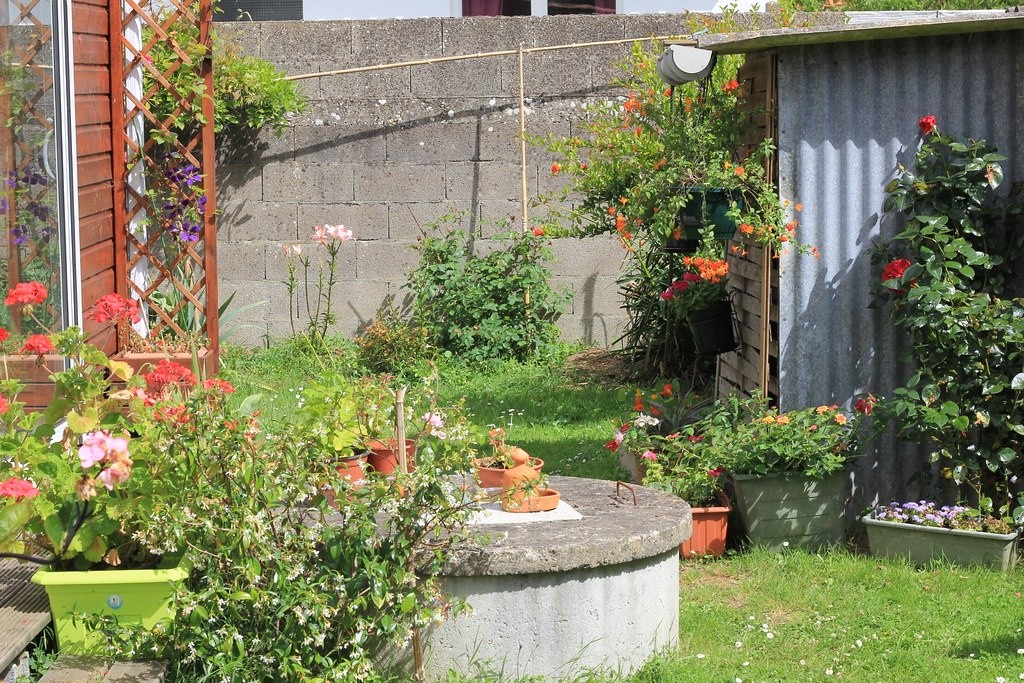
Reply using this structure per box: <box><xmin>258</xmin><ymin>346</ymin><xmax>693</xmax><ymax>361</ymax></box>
<box><xmin>469</xmin><ymin>456</ymin><xmax>544</xmax><ymax>488</ymax></box>
<box><xmin>679</xmin><ymin>486</ymin><xmax>734</xmax><ymax>560</ymax></box>
<box><xmin>501</xmin><ymin>447</ymin><xmax>560</xmax><ymax>512</ymax></box>
<box><xmin>685</xmin><ymin>300</ymin><xmax>737</xmax><ymax>357</ymax></box>
<box><xmin>729</xmin><ymin>463</ymin><xmax>848</xmax><ymax>552</ymax></box>
<box><xmin>123</xmin><ymin>351</ymin><xmax>208</xmax><ymax>383</ymax></box>
<box><xmin>862</xmin><ymin>505</ymin><xmax>1024</xmax><ymax>575</ymax></box>
<box><xmin>665</xmin><ymin>184</ymin><xmax>743</xmax><ymax>239</ymax></box>
<box><xmin>618</xmin><ymin>444</ymin><xmax>665</xmax><ymax>486</ymax></box>
<box><xmin>655</xmin><ymin>216</ymin><xmax>702</xmax><ymax>251</ymax></box>
<box><xmin>31</xmin><ymin>543</ymin><xmax>200</xmax><ymax>659</ymax></box>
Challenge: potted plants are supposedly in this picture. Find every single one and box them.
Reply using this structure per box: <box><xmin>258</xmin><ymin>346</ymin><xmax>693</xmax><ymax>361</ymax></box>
<box><xmin>302</xmin><ymin>368</ymin><xmax>370</xmax><ymax>505</ymax></box>
<box><xmin>365</xmin><ymin>375</ymin><xmax>418</xmax><ymax>480</ymax></box>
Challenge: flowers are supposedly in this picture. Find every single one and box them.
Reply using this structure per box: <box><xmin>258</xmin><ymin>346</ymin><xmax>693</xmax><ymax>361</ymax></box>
<box><xmin>869</xmin><ymin>500</ymin><xmax>1014</xmax><ymax>534</ymax></box>
<box><xmin>521</xmin><ymin>37</ymin><xmax>819</xmax><ymax>282</ymax></box>
<box><xmin>660</xmin><ymin>256</ymin><xmax>732</xmax><ymax>324</ymax></box>
<box><xmin>605</xmin><ymin>410</ymin><xmax>660</xmax><ymax>454</ymax></box>
<box><xmin>0</xmin><ymin>282</ymin><xmax>231</xmax><ymax>568</ymax></box>
<box><xmin>638</xmin><ymin>431</ymin><xmax>725</xmax><ymax>506</ymax></box>
<box><xmin>710</xmin><ymin>388</ymin><xmax>875</xmax><ymax>478</ymax></box>
<box><xmin>479</xmin><ymin>426</ymin><xmax>535</xmax><ymax>469</ymax></box>
<box><xmin>118</xmin><ymin>321</ymin><xmax>210</xmax><ymax>354</ymax></box>
